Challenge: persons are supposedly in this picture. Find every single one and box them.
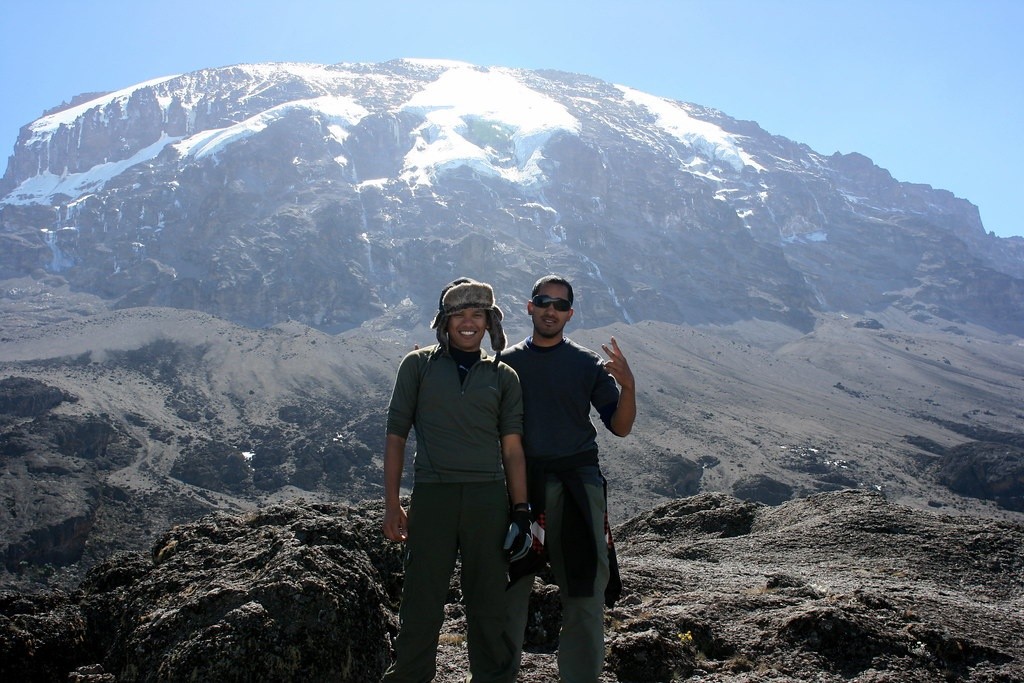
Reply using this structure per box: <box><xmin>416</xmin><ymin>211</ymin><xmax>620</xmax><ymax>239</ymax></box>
<box><xmin>488</xmin><ymin>274</ymin><xmax>635</xmax><ymax>683</ymax></box>
<box><xmin>383</xmin><ymin>276</ymin><xmax>531</xmax><ymax>682</ymax></box>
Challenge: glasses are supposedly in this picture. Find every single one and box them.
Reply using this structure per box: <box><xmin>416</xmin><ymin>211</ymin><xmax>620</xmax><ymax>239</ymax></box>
<box><xmin>531</xmin><ymin>295</ymin><xmax>571</xmax><ymax>312</ymax></box>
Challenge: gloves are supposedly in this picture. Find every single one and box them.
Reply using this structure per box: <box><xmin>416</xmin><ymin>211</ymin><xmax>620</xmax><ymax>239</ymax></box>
<box><xmin>504</xmin><ymin>505</ymin><xmax>533</xmax><ymax>563</ymax></box>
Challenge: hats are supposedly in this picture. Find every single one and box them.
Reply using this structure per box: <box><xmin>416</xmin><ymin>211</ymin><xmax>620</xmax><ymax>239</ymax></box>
<box><xmin>430</xmin><ymin>277</ymin><xmax>506</xmax><ymax>369</ymax></box>
